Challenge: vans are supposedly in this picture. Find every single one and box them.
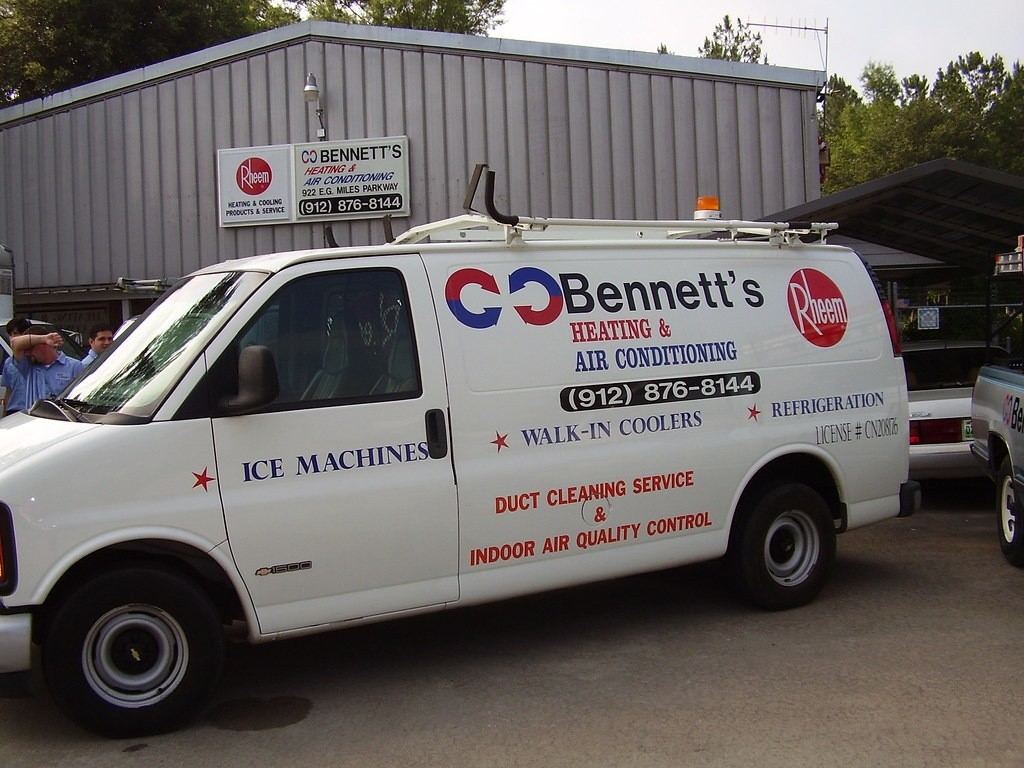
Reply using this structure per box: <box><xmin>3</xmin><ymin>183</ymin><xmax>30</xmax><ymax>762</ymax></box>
<box><xmin>0</xmin><ymin>164</ymin><xmax>917</xmax><ymax>739</ymax></box>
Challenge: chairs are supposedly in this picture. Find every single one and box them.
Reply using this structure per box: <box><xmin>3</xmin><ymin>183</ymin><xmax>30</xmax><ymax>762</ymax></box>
<box><xmin>301</xmin><ymin>303</ymin><xmax>361</xmax><ymax>401</ymax></box>
<box><xmin>368</xmin><ymin>305</ymin><xmax>420</xmax><ymax>397</ymax></box>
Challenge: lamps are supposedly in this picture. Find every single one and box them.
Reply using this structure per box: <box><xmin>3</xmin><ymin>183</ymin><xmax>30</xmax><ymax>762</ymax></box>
<box><xmin>304</xmin><ymin>72</ymin><xmax>323</xmax><ymax>114</ymax></box>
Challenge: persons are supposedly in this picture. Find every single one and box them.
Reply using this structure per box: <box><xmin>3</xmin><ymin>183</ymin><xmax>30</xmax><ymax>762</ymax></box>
<box><xmin>81</xmin><ymin>323</ymin><xmax>114</xmax><ymax>366</ymax></box>
<box><xmin>0</xmin><ymin>317</ymin><xmax>85</xmax><ymax>420</ymax></box>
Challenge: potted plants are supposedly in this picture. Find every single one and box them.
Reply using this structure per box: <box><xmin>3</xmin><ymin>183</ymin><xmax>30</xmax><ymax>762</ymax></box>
<box><xmin>920</xmin><ymin>288</ymin><xmax>946</xmax><ymax>306</ymax></box>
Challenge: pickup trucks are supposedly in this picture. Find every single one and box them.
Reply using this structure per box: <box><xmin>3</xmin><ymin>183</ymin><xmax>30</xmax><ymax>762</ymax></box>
<box><xmin>970</xmin><ymin>362</ymin><xmax>1024</xmax><ymax>568</ymax></box>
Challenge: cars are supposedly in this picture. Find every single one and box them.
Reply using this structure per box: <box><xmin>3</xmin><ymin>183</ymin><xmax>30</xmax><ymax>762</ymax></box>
<box><xmin>900</xmin><ymin>339</ymin><xmax>1014</xmax><ymax>483</ymax></box>
